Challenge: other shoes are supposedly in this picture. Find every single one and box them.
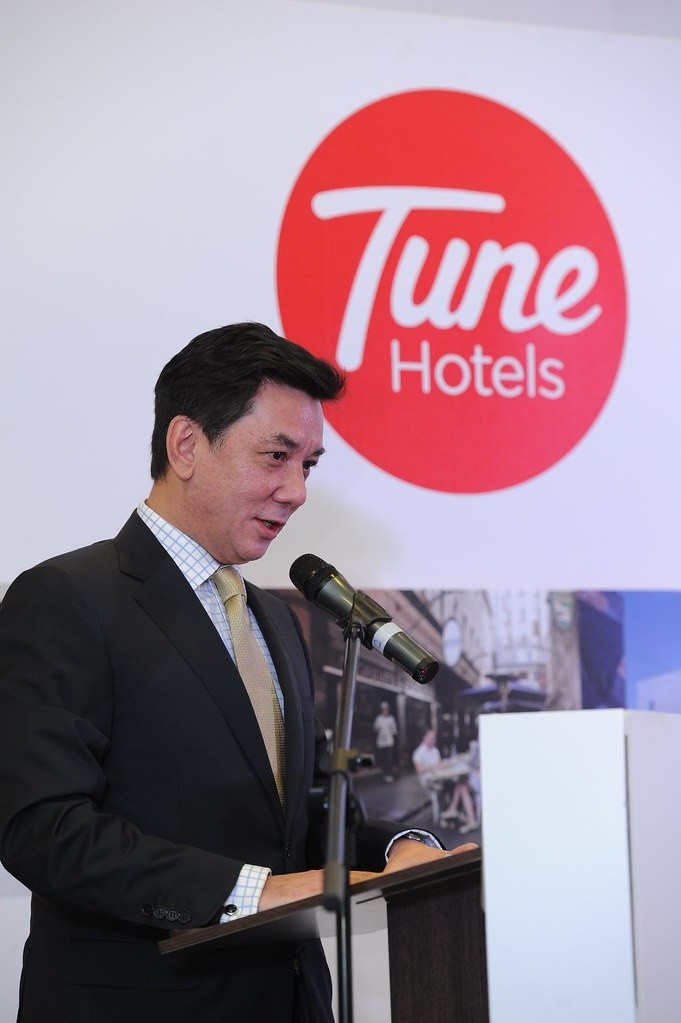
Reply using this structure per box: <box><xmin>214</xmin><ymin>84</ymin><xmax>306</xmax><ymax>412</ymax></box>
<box><xmin>459</xmin><ymin>822</ymin><xmax>479</xmax><ymax>835</ymax></box>
<box><xmin>441</xmin><ymin>810</ymin><xmax>460</xmax><ymax>819</ymax></box>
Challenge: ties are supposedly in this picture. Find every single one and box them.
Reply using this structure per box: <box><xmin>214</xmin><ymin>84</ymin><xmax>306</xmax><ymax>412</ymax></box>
<box><xmin>213</xmin><ymin>565</ymin><xmax>288</xmax><ymax>815</ymax></box>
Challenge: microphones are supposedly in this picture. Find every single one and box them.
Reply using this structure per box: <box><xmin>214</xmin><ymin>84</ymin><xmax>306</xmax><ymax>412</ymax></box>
<box><xmin>289</xmin><ymin>553</ymin><xmax>440</xmax><ymax>684</ymax></box>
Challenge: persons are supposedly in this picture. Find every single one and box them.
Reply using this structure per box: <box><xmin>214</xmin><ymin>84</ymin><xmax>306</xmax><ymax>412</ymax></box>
<box><xmin>374</xmin><ymin>699</ymin><xmax>401</xmax><ymax>783</ymax></box>
<box><xmin>414</xmin><ymin>728</ymin><xmax>482</xmax><ymax>834</ymax></box>
<box><xmin>0</xmin><ymin>322</ymin><xmax>479</xmax><ymax>1023</ymax></box>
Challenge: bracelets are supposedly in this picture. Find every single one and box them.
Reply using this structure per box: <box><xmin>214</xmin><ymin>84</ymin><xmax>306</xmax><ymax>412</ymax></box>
<box><xmin>404</xmin><ymin>833</ymin><xmax>421</xmax><ymax>840</ymax></box>
